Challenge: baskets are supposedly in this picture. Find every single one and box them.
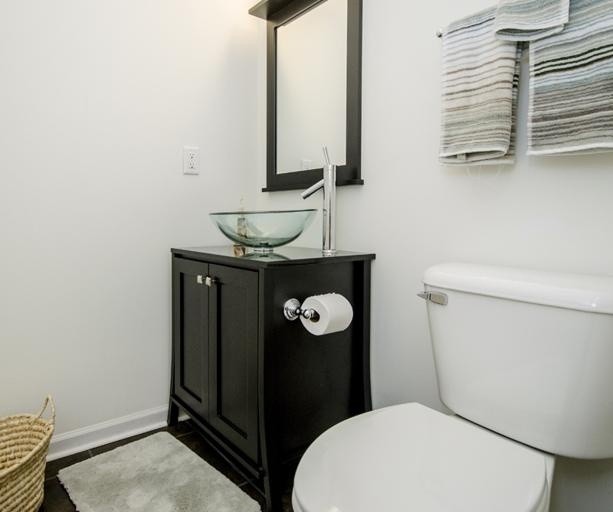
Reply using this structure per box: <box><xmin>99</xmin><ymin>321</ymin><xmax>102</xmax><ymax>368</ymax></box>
<box><xmin>1</xmin><ymin>397</ymin><xmax>55</xmax><ymax>511</ymax></box>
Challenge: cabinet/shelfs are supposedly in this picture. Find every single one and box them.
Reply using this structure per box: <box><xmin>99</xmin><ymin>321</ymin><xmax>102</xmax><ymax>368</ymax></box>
<box><xmin>165</xmin><ymin>248</ymin><xmax>376</xmax><ymax>510</ymax></box>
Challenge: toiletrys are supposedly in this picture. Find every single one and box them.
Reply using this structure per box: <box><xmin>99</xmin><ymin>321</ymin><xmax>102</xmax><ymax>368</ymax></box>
<box><xmin>233</xmin><ymin>218</ymin><xmax>248</xmax><ymax>258</ymax></box>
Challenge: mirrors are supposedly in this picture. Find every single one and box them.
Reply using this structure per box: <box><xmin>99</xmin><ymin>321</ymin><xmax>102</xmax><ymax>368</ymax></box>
<box><xmin>247</xmin><ymin>1</ymin><xmax>364</xmax><ymax>192</ymax></box>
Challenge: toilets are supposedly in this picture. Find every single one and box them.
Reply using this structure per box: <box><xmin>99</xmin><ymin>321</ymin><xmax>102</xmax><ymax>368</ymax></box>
<box><xmin>290</xmin><ymin>264</ymin><xmax>613</xmax><ymax>512</ymax></box>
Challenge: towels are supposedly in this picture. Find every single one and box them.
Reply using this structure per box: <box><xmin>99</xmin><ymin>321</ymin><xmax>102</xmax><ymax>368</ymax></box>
<box><xmin>437</xmin><ymin>0</ymin><xmax>613</xmax><ymax>167</ymax></box>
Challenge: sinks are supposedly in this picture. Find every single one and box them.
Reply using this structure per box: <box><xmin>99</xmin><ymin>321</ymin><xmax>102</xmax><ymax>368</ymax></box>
<box><xmin>208</xmin><ymin>209</ymin><xmax>317</xmax><ymax>248</ymax></box>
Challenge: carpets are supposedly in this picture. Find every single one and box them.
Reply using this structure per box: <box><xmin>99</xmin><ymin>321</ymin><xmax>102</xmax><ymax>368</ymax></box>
<box><xmin>55</xmin><ymin>429</ymin><xmax>262</xmax><ymax>510</ymax></box>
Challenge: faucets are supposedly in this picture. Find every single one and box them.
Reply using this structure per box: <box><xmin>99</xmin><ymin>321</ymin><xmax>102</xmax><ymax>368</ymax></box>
<box><xmin>302</xmin><ymin>145</ymin><xmax>336</xmax><ymax>253</ymax></box>
